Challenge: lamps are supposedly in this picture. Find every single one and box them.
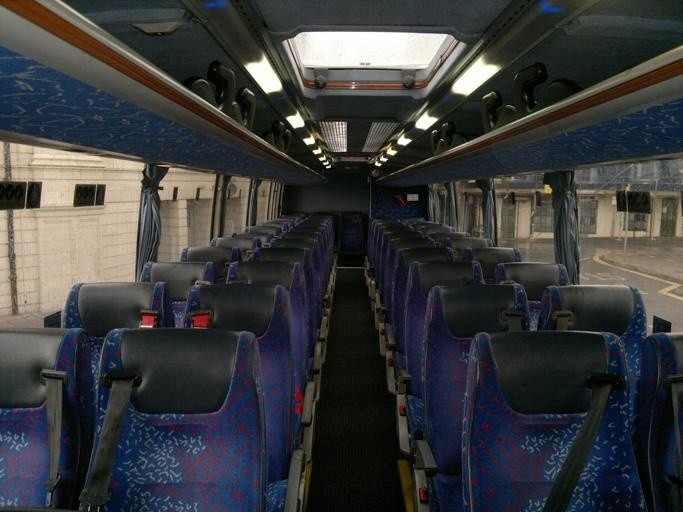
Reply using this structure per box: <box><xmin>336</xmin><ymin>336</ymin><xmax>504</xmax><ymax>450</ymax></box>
<box><xmin>197</xmin><ymin>1</ymin><xmax>333</xmax><ymax>171</ymax></box>
<box><xmin>317</xmin><ymin>116</ymin><xmax>400</xmax><ymax>155</ymax></box>
<box><xmin>370</xmin><ymin>0</ymin><xmax>599</xmax><ymax>168</ymax></box>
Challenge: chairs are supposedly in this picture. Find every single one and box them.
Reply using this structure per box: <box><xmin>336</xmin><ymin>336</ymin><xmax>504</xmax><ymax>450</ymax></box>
<box><xmin>0</xmin><ymin>209</ymin><xmax>682</xmax><ymax>512</ymax></box>
